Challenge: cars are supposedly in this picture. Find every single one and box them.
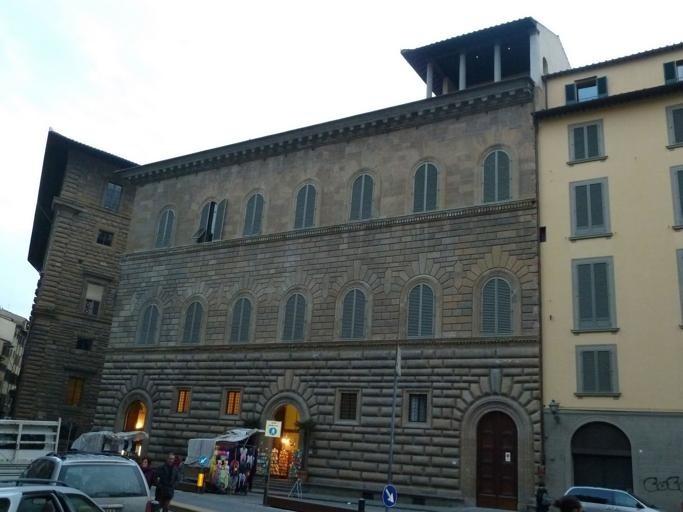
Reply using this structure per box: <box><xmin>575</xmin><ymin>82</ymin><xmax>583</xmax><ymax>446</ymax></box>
<box><xmin>0</xmin><ymin>419</ymin><xmax>154</xmax><ymax>512</ymax></box>
<box><xmin>562</xmin><ymin>486</ymin><xmax>659</xmax><ymax>512</ymax></box>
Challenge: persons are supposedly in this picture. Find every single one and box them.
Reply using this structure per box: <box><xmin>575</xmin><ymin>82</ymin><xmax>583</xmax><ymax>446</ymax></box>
<box><xmin>139</xmin><ymin>456</ymin><xmax>156</xmax><ymax>489</ymax></box>
<box><xmin>154</xmin><ymin>453</ymin><xmax>181</xmax><ymax>512</ymax></box>
<box><xmin>553</xmin><ymin>495</ymin><xmax>582</xmax><ymax>512</ymax></box>
